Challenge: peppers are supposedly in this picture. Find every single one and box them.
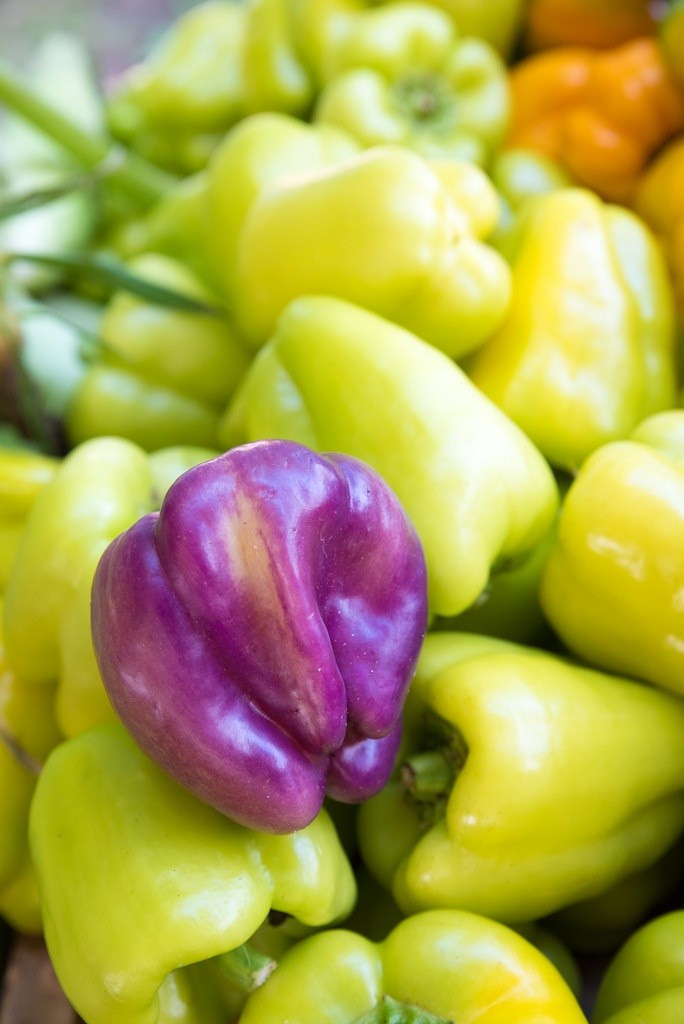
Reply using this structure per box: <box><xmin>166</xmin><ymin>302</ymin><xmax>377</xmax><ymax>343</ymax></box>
<box><xmin>0</xmin><ymin>0</ymin><xmax>684</xmax><ymax>1024</ymax></box>
